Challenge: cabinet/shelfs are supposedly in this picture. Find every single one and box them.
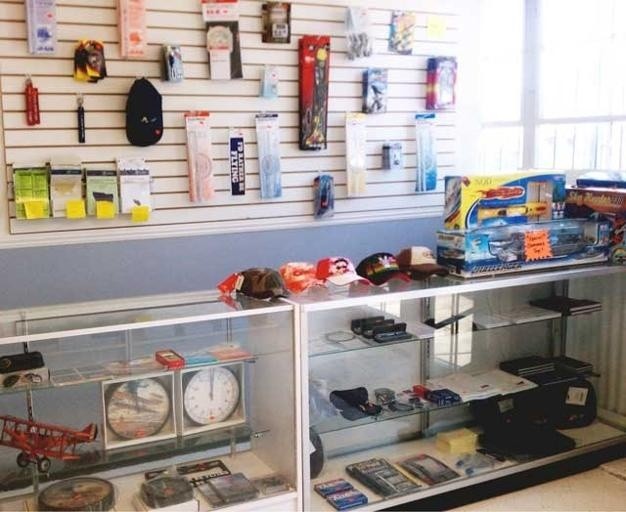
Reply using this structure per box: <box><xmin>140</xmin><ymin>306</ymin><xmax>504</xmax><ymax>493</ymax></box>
<box><xmin>2</xmin><ymin>266</ymin><xmax>624</xmax><ymax>512</ymax></box>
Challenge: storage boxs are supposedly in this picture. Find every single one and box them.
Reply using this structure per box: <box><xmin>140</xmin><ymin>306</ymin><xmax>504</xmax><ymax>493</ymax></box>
<box><xmin>435</xmin><ymin>170</ymin><xmax>625</xmax><ymax>279</ymax></box>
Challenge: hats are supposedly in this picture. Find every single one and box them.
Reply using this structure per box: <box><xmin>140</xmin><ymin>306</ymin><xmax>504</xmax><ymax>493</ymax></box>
<box><xmin>279</xmin><ymin>262</ymin><xmax>328</xmax><ymax>297</ymax></box>
<box><xmin>236</xmin><ymin>267</ymin><xmax>291</xmax><ymax>301</ymax></box>
<box><xmin>355</xmin><ymin>253</ymin><xmax>410</xmax><ymax>286</ymax></box>
<box><xmin>126</xmin><ymin>78</ymin><xmax>162</xmax><ymax>146</ymax></box>
<box><xmin>398</xmin><ymin>246</ymin><xmax>449</xmax><ymax>280</ymax></box>
<box><xmin>317</xmin><ymin>257</ymin><xmax>374</xmax><ymax>287</ymax></box>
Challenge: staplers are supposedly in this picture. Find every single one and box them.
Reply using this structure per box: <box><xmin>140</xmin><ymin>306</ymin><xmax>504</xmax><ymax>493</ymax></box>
<box><xmin>350</xmin><ymin>316</ymin><xmax>412</xmax><ymax>343</ymax></box>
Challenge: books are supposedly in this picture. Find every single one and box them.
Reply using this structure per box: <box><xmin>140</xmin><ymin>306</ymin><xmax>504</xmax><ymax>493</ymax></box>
<box><xmin>425</xmin><ymin>297</ymin><xmax>603</xmax><ymax>403</ymax></box>
